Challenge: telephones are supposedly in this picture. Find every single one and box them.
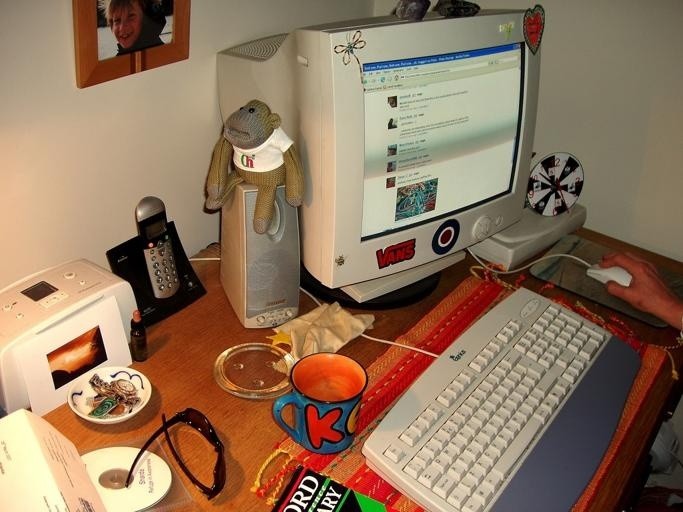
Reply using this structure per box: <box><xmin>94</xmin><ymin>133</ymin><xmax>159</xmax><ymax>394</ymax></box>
<box><xmin>106</xmin><ymin>196</ymin><xmax>206</xmax><ymax>328</ymax></box>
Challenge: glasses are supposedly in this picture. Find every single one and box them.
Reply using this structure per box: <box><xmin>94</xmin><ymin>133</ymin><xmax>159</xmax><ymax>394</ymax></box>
<box><xmin>125</xmin><ymin>409</ymin><xmax>227</xmax><ymax>501</ymax></box>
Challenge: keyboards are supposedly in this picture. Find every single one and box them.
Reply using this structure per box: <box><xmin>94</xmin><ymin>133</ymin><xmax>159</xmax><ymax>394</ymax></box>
<box><xmin>361</xmin><ymin>285</ymin><xmax>613</xmax><ymax>512</ymax></box>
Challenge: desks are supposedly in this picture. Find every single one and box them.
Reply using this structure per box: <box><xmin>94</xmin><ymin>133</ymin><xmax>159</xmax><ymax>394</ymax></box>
<box><xmin>2</xmin><ymin>234</ymin><xmax>682</xmax><ymax>512</ymax></box>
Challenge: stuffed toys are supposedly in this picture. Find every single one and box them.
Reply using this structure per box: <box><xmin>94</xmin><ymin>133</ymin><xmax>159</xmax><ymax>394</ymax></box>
<box><xmin>204</xmin><ymin>99</ymin><xmax>305</xmax><ymax>233</ymax></box>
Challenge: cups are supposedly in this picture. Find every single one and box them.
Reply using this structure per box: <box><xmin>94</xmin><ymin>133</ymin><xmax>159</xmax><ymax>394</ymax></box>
<box><xmin>272</xmin><ymin>352</ymin><xmax>368</xmax><ymax>454</ymax></box>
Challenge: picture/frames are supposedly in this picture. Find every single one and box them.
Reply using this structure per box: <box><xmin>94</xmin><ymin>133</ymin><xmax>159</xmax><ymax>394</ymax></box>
<box><xmin>73</xmin><ymin>0</ymin><xmax>190</xmax><ymax>90</ymax></box>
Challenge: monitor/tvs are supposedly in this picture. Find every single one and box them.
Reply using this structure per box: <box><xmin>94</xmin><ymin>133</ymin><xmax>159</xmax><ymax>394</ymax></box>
<box><xmin>215</xmin><ymin>11</ymin><xmax>541</xmax><ymax>304</ymax></box>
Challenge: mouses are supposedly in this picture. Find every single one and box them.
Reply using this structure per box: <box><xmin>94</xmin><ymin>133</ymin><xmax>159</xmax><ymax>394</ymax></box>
<box><xmin>586</xmin><ymin>262</ymin><xmax>632</xmax><ymax>287</ymax></box>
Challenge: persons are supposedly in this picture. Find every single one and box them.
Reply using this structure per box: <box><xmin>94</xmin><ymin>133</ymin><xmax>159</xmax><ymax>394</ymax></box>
<box><xmin>98</xmin><ymin>0</ymin><xmax>166</xmax><ymax>57</ymax></box>
<box><xmin>596</xmin><ymin>249</ymin><xmax>683</xmax><ymax>331</ymax></box>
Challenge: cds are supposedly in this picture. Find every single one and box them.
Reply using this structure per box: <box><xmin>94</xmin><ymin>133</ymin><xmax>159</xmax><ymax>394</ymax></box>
<box><xmin>78</xmin><ymin>445</ymin><xmax>172</xmax><ymax>511</ymax></box>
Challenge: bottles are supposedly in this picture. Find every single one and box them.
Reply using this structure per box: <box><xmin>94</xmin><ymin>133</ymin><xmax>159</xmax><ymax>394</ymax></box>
<box><xmin>131</xmin><ymin>311</ymin><xmax>147</xmax><ymax>362</ymax></box>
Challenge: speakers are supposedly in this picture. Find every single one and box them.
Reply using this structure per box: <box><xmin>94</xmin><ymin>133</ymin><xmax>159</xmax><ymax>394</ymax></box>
<box><xmin>220</xmin><ymin>178</ymin><xmax>299</xmax><ymax>330</ymax></box>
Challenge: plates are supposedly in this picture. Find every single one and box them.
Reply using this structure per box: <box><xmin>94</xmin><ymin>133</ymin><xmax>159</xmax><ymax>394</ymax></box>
<box><xmin>70</xmin><ymin>366</ymin><xmax>151</xmax><ymax>425</ymax></box>
